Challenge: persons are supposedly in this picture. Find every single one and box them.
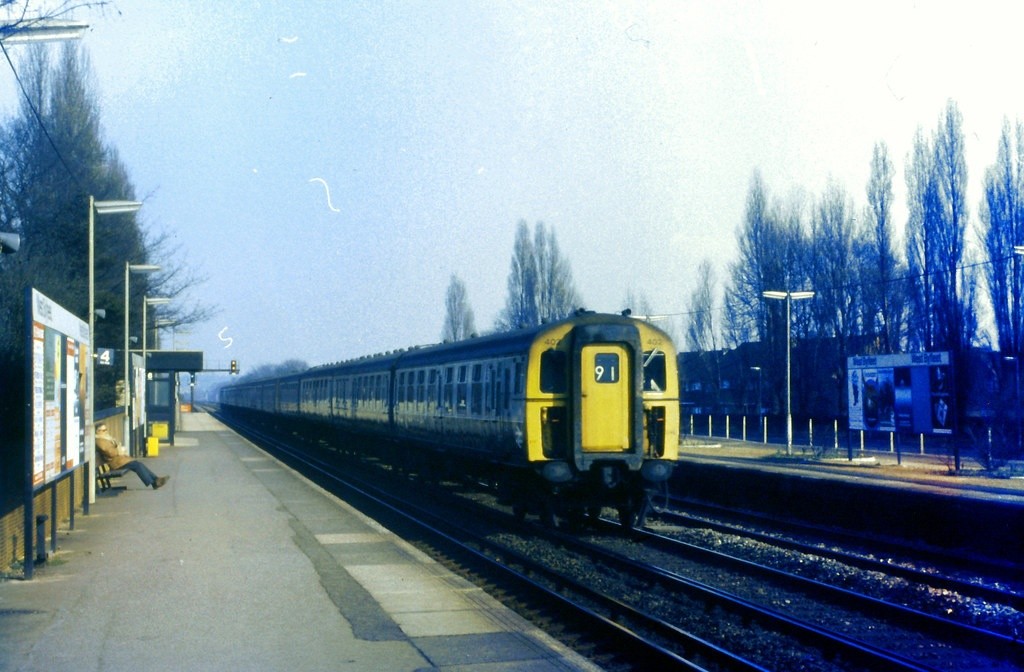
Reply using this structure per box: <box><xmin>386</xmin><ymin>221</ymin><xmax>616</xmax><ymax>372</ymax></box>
<box><xmin>851</xmin><ymin>371</ymin><xmax>859</xmax><ymax>406</ymax></box>
<box><xmin>95</xmin><ymin>422</ymin><xmax>170</xmax><ymax>489</ymax></box>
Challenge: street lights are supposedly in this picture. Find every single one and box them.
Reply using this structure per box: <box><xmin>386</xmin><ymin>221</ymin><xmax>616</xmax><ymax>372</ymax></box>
<box><xmin>171</xmin><ymin>330</ymin><xmax>193</xmax><ymax>431</ymax></box>
<box><xmin>87</xmin><ymin>195</ymin><xmax>141</xmax><ymax>514</ymax></box>
<box><xmin>138</xmin><ymin>294</ymin><xmax>171</xmax><ymax>453</ymax></box>
<box><xmin>762</xmin><ymin>291</ymin><xmax>815</xmax><ymax>455</ymax></box>
<box><xmin>123</xmin><ymin>260</ymin><xmax>158</xmax><ymax>450</ymax></box>
<box><xmin>152</xmin><ymin>317</ymin><xmax>172</xmax><ymax>404</ymax></box>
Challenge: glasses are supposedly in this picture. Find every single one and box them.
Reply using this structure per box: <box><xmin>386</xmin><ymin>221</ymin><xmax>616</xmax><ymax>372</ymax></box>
<box><xmin>98</xmin><ymin>429</ymin><xmax>107</xmax><ymax>432</ymax></box>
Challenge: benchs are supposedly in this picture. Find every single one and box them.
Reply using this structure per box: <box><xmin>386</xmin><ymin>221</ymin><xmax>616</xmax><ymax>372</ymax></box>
<box><xmin>96</xmin><ymin>451</ymin><xmax>130</xmax><ymax>493</ymax></box>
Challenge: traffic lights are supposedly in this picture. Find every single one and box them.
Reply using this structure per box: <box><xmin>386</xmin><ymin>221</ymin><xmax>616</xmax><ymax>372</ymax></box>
<box><xmin>230</xmin><ymin>360</ymin><xmax>237</xmax><ymax>373</ymax></box>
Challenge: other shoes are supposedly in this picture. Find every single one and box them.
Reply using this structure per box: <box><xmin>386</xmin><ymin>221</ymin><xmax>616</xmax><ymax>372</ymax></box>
<box><xmin>152</xmin><ymin>475</ymin><xmax>170</xmax><ymax>489</ymax></box>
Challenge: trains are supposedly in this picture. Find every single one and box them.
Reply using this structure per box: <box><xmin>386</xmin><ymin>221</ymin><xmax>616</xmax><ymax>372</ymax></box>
<box><xmin>221</xmin><ymin>308</ymin><xmax>683</xmax><ymax>533</ymax></box>
